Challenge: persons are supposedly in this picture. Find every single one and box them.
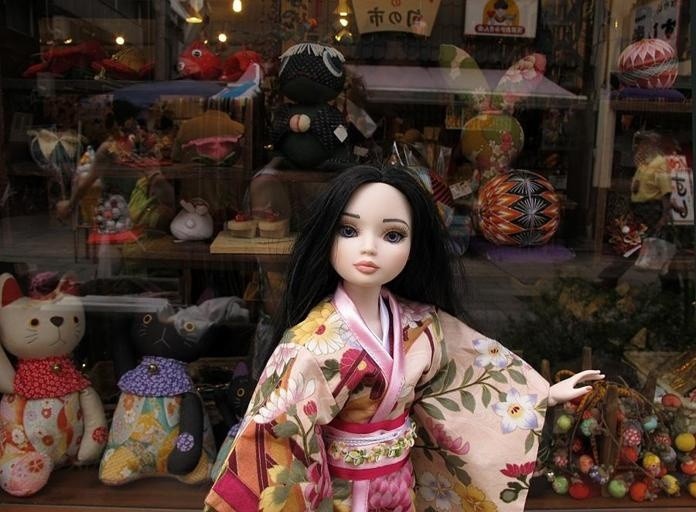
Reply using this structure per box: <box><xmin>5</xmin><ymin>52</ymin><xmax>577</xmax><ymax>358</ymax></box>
<box><xmin>207</xmin><ymin>166</ymin><xmax>605</xmax><ymax>512</ymax></box>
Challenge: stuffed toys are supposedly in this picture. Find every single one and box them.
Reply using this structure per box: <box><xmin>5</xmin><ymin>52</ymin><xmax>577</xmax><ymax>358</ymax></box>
<box><xmin>0</xmin><ymin>267</ymin><xmax>219</xmax><ymax>498</ymax></box>
